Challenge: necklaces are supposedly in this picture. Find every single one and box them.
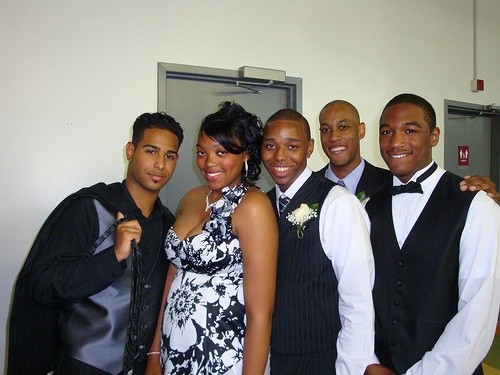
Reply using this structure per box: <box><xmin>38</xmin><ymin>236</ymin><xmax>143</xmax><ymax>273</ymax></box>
<box><xmin>204</xmin><ymin>189</ymin><xmax>223</xmax><ymax>212</ymax></box>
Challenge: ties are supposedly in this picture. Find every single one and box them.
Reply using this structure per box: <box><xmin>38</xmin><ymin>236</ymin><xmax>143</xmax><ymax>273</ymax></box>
<box><xmin>279</xmin><ymin>196</ymin><xmax>290</xmax><ymax>214</ymax></box>
<box><xmin>337</xmin><ymin>181</ymin><xmax>345</xmax><ymax>187</ymax></box>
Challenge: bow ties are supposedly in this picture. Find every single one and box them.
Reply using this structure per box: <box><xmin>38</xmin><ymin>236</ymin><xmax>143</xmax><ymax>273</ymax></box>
<box><xmin>392</xmin><ymin>162</ymin><xmax>437</xmax><ymax>196</ymax></box>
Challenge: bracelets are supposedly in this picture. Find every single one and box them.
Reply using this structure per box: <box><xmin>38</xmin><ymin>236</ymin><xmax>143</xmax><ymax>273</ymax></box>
<box><xmin>145</xmin><ymin>351</ymin><xmax>161</xmax><ymax>355</ymax></box>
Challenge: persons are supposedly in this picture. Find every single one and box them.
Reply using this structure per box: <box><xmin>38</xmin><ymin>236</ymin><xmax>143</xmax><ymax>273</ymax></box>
<box><xmin>145</xmin><ymin>101</ymin><xmax>279</xmax><ymax>375</ymax></box>
<box><xmin>315</xmin><ymin>99</ymin><xmax>500</xmax><ymax>205</ymax></box>
<box><xmin>262</xmin><ymin>109</ymin><xmax>375</xmax><ymax>375</ymax></box>
<box><xmin>365</xmin><ymin>93</ymin><xmax>500</xmax><ymax>374</ymax></box>
<box><xmin>4</xmin><ymin>112</ymin><xmax>185</xmax><ymax>375</ymax></box>
